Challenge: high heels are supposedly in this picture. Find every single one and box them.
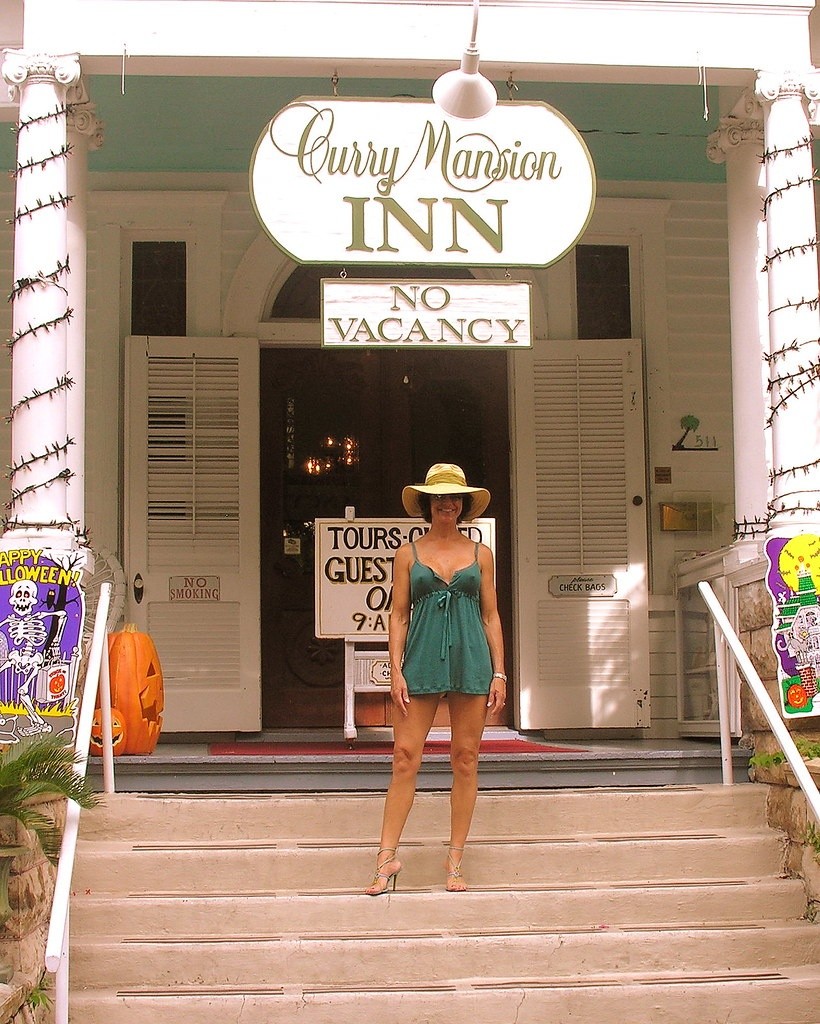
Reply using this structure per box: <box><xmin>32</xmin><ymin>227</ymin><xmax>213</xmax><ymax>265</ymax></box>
<box><xmin>365</xmin><ymin>848</ymin><xmax>401</xmax><ymax>895</ymax></box>
<box><xmin>445</xmin><ymin>847</ymin><xmax>466</xmax><ymax>892</ymax></box>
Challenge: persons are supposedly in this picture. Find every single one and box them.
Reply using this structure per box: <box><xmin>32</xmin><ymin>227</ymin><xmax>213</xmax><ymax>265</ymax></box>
<box><xmin>363</xmin><ymin>463</ymin><xmax>506</xmax><ymax>895</ymax></box>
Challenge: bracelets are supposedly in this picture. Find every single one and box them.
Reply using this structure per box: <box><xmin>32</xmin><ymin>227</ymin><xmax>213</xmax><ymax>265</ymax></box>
<box><xmin>493</xmin><ymin>672</ymin><xmax>506</xmax><ymax>683</ymax></box>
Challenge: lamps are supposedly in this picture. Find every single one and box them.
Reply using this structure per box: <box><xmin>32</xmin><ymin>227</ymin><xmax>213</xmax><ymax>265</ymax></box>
<box><xmin>432</xmin><ymin>0</ymin><xmax>498</xmax><ymax>119</ymax></box>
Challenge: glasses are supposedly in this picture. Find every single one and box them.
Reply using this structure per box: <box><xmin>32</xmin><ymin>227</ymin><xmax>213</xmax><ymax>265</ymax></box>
<box><xmin>430</xmin><ymin>495</ymin><xmax>462</xmax><ymax>501</ymax></box>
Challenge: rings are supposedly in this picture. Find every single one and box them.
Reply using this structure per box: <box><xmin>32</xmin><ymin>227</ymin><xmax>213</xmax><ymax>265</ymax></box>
<box><xmin>502</xmin><ymin>702</ymin><xmax>505</xmax><ymax>706</ymax></box>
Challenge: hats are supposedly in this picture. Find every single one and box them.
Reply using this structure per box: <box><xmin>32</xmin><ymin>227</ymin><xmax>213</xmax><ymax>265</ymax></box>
<box><xmin>402</xmin><ymin>463</ymin><xmax>492</xmax><ymax>521</ymax></box>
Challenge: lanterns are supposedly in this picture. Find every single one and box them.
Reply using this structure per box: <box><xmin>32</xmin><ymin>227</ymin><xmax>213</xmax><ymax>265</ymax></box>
<box><xmin>90</xmin><ymin>624</ymin><xmax>164</xmax><ymax>756</ymax></box>
